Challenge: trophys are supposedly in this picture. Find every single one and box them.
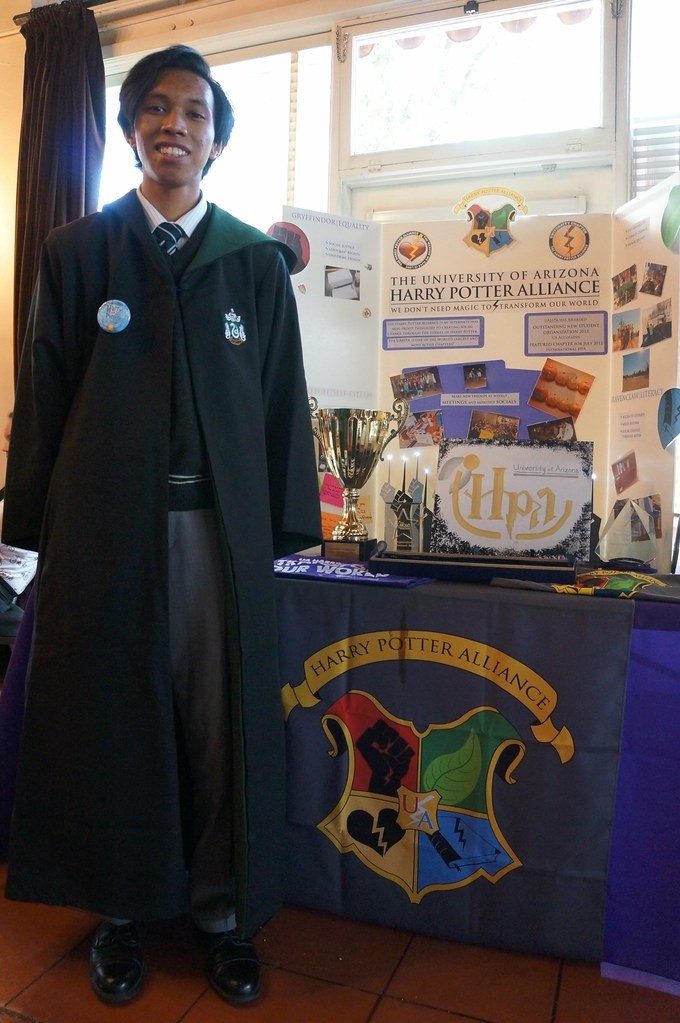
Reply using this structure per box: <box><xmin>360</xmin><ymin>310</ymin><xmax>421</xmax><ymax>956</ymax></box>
<box><xmin>308</xmin><ymin>396</ymin><xmax>409</xmax><ymax>560</ymax></box>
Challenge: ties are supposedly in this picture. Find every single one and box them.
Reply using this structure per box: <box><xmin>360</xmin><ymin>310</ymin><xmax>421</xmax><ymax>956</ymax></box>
<box><xmin>155</xmin><ymin>223</ymin><xmax>183</xmax><ymax>256</ymax></box>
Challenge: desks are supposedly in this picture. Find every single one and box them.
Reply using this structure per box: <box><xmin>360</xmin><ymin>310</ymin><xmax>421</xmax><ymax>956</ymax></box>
<box><xmin>273</xmin><ymin>574</ymin><xmax>679</xmax><ymax>996</ymax></box>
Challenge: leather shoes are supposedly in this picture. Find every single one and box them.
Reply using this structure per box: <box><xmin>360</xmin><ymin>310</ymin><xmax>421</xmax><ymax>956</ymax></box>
<box><xmin>90</xmin><ymin>920</ymin><xmax>143</xmax><ymax>1003</ymax></box>
<box><xmin>204</xmin><ymin>931</ymin><xmax>265</xmax><ymax>1009</ymax></box>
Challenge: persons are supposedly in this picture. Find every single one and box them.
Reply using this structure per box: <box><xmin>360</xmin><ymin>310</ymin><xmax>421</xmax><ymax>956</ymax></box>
<box><xmin>471</xmin><ymin>418</ymin><xmax>517</xmax><ymax>439</ymax></box>
<box><xmin>649</xmin><ymin>319</ymin><xmax>667</xmax><ymax>339</ymax></box>
<box><xmin>623</xmin><ymin>326</ymin><xmax>629</xmax><ymax>347</ymax></box>
<box><xmin>556</xmin><ymin>421</ymin><xmax>573</xmax><ymax>442</ymax></box>
<box><xmin>0</xmin><ymin>45</ymin><xmax>323</xmax><ymax>1006</ymax></box>
<box><xmin>391</xmin><ymin>371</ymin><xmax>438</xmax><ymax>398</ymax></box>
<box><xmin>469</xmin><ymin>369</ymin><xmax>482</xmax><ymax>381</ymax></box>
